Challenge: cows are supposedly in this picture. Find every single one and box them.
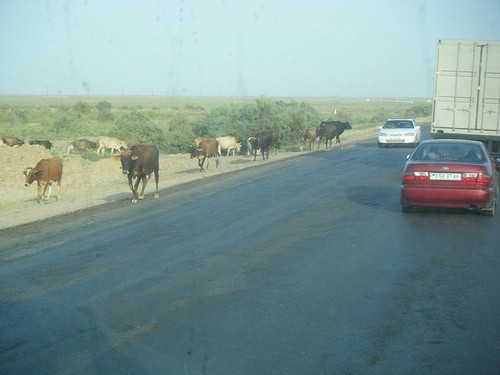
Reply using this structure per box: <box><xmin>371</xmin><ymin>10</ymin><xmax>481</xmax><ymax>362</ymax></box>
<box><xmin>252</xmin><ymin>130</ymin><xmax>279</xmax><ymax>154</ymax></box>
<box><xmin>245</xmin><ymin>136</ymin><xmax>261</xmax><ymax>156</ymax></box>
<box><xmin>73</xmin><ymin>139</ymin><xmax>98</xmax><ymax>153</ymax></box>
<box><xmin>96</xmin><ymin>136</ymin><xmax>128</xmax><ymax>156</ymax></box>
<box><xmin>23</xmin><ymin>156</ymin><xmax>63</xmax><ymax>204</ymax></box>
<box><xmin>215</xmin><ymin>135</ymin><xmax>242</xmax><ymax>157</ymax></box>
<box><xmin>67</xmin><ymin>143</ymin><xmax>76</xmax><ymax>155</ymax></box>
<box><xmin>248</xmin><ymin>131</ymin><xmax>273</xmax><ymax>161</ymax></box>
<box><xmin>319</xmin><ymin>120</ymin><xmax>352</xmax><ymax>143</ymax></box>
<box><xmin>195</xmin><ymin>136</ymin><xmax>219</xmax><ymax>172</ymax></box>
<box><xmin>316</xmin><ymin>124</ymin><xmax>339</xmax><ymax>152</ymax></box>
<box><xmin>28</xmin><ymin>140</ymin><xmax>52</xmax><ymax>150</ymax></box>
<box><xmin>2</xmin><ymin>136</ymin><xmax>25</xmax><ymax>147</ymax></box>
<box><xmin>303</xmin><ymin>127</ymin><xmax>318</xmax><ymax>151</ymax></box>
<box><xmin>114</xmin><ymin>144</ymin><xmax>160</xmax><ymax>203</ymax></box>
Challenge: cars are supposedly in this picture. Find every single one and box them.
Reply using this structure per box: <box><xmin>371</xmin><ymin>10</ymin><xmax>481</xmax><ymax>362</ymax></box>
<box><xmin>401</xmin><ymin>139</ymin><xmax>500</xmax><ymax>216</ymax></box>
<box><xmin>378</xmin><ymin>118</ymin><xmax>420</xmax><ymax>148</ymax></box>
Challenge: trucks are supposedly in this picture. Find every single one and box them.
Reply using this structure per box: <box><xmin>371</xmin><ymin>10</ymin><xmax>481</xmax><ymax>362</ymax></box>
<box><xmin>431</xmin><ymin>39</ymin><xmax>500</xmax><ymax>156</ymax></box>
